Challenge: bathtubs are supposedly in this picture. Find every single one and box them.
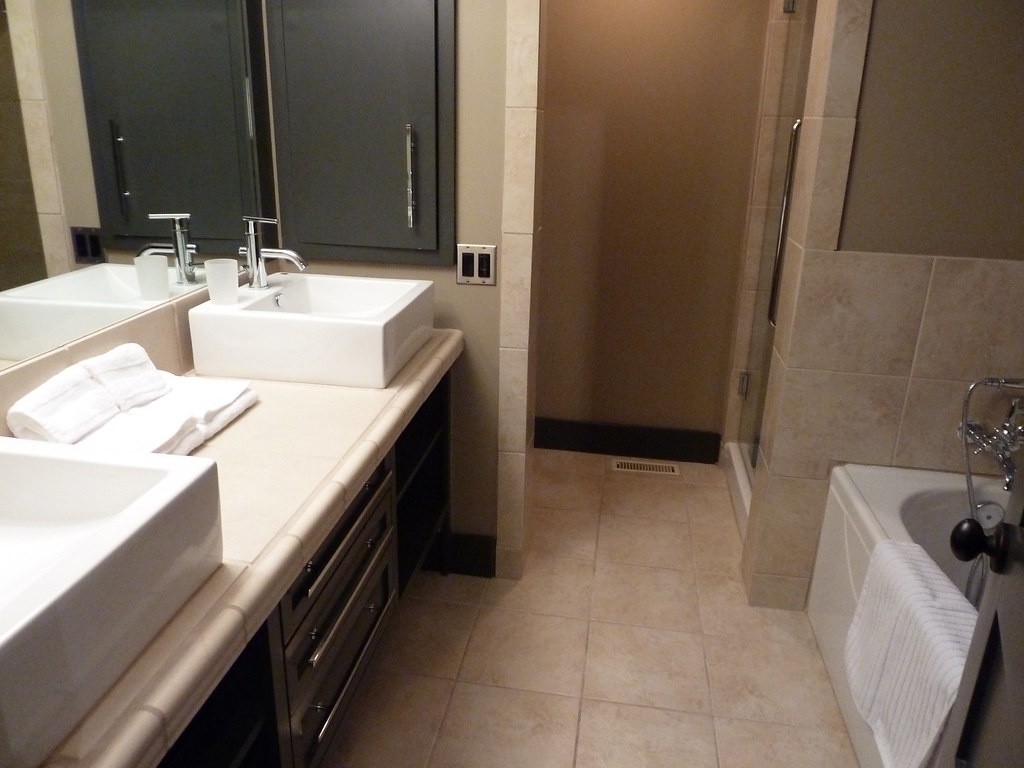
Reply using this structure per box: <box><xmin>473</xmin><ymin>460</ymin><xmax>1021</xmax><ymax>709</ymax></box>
<box><xmin>805</xmin><ymin>465</ymin><xmax>1022</xmax><ymax>768</ymax></box>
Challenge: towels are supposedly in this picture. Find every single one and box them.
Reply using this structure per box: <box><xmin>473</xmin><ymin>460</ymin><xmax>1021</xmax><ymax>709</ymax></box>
<box><xmin>6</xmin><ymin>344</ymin><xmax>260</xmax><ymax>457</ymax></box>
<box><xmin>845</xmin><ymin>541</ymin><xmax>980</xmax><ymax>768</ymax></box>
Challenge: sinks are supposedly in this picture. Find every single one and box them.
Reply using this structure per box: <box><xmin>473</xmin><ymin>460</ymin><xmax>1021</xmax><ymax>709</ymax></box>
<box><xmin>1</xmin><ymin>299</ymin><xmax>141</xmax><ymax>370</ymax></box>
<box><xmin>2</xmin><ymin>437</ymin><xmax>223</xmax><ymax>767</ymax></box>
<box><xmin>190</xmin><ymin>271</ymin><xmax>431</xmax><ymax>387</ymax></box>
<box><xmin>8</xmin><ymin>262</ymin><xmax>234</xmax><ymax>310</ymax></box>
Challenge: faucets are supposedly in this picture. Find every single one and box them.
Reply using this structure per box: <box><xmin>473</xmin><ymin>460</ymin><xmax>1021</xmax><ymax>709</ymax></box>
<box><xmin>239</xmin><ymin>214</ymin><xmax>309</xmax><ymax>289</ymax></box>
<box><xmin>133</xmin><ymin>213</ymin><xmax>200</xmax><ymax>286</ymax></box>
<box><xmin>960</xmin><ymin>401</ymin><xmax>1024</xmax><ymax>491</ymax></box>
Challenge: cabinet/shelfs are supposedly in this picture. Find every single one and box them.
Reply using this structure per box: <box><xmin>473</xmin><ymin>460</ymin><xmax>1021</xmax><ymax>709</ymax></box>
<box><xmin>264</xmin><ymin>0</ymin><xmax>458</xmax><ymax>266</ymax></box>
<box><xmin>156</xmin><ymin>370</ymin><xmax>452</xmax><ymax>768</ymax></box>
<box><xmin>71</xmin><ymin>0</ymin><xmax>265</xmax><ymax>254</ymax></box>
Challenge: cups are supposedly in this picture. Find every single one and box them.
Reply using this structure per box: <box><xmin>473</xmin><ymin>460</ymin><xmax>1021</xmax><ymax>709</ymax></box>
<box><xmin>204</xmin><ymin>259</ymin><xmax>239</xmax><ymax>306</ymax></box>
<box><xmin>135</xmin><ymin>255</ymin><xmax>167</xmax><ymax>300</ymax></box>
